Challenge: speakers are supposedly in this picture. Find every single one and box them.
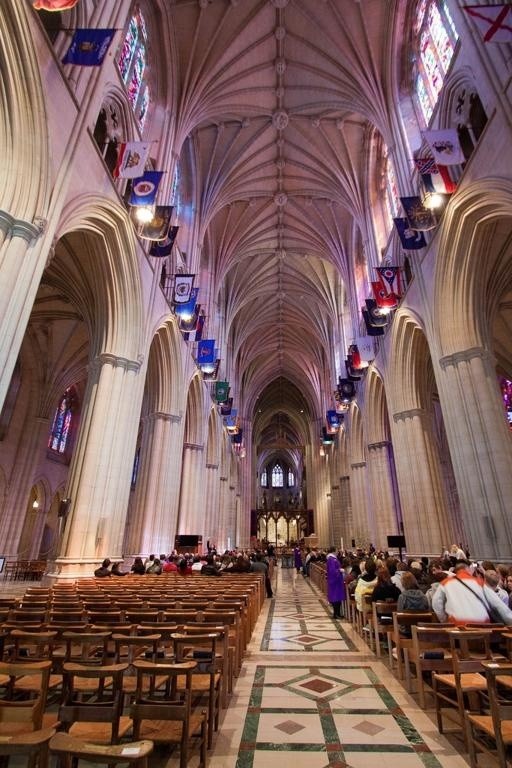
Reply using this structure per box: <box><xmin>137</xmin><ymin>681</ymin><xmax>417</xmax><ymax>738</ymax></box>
<box><xmin>387</xmin><ymin>536</ymin><xmax>406</xmax><ymax>548</ymax></box>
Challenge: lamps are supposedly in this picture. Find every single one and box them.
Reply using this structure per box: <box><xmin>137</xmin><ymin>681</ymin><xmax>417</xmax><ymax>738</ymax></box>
<box><xmin>416</xmin><ymin>168</ymin><xmax>444</xmax><ymax>210</ymax></box>
<box><xmin>135</xmin><ymin>191</ymin><xmax>156</xmax><ymax>224</ymax></box>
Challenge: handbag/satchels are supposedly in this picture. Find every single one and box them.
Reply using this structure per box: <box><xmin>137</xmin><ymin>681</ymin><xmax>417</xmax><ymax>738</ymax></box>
<box><xmin>489</xmin><ymin>608</ymin><xmax>504</xmax><ymax>625</ymax></box>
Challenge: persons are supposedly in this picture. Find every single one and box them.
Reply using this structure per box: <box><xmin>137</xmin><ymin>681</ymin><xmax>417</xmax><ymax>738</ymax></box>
<box><xmin>294</xmin><ymin>542</ymin><xmax>512</xmax><ymax>662</ymax></box>
<box><xmin>94</xmin><ymin>537</ymin><xmax>277</xmax><ymax>598</ymax></box>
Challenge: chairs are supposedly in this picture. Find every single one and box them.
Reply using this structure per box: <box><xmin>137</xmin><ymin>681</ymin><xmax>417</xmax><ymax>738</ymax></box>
<box><xmin>309</xmin><ymin>549</ymin><xmax>512</xmax><ymax>768</ymax></box>
<box><xmin>0</xmin><ymin>567</ymin><xmax>269</xmax><ymax>768</ymax></box>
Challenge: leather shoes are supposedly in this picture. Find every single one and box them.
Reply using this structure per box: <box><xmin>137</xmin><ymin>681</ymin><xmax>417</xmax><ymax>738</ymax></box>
<box><xmin>331</xmin><ymin>613</ymin><xmax>344</xmax><ymax>618</ymax></box>
